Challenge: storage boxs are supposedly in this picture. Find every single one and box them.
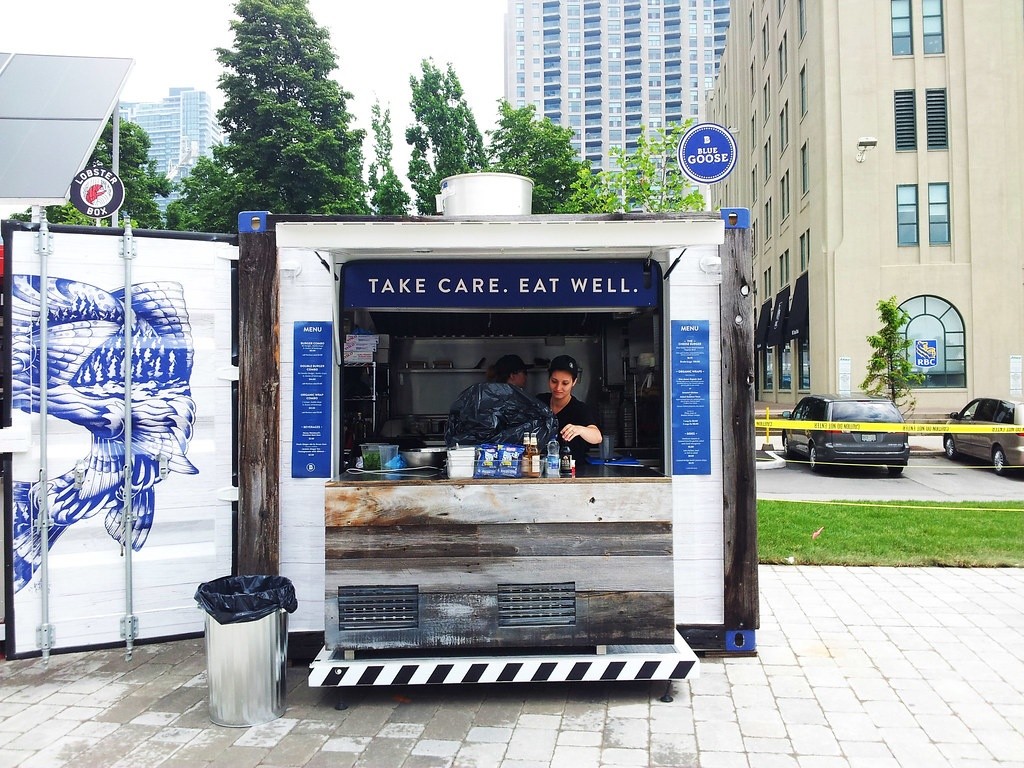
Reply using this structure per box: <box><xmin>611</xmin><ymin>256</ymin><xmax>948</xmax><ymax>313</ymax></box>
<box><xmin>343</xmin><ymin>334</ymin><xmax>391</xmax><ymax>363</ymax></box>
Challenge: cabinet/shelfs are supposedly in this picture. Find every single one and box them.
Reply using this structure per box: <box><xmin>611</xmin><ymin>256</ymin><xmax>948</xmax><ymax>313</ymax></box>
<box><xmin>343</xmin><ymin>363</ymin><xmax>390</xmax><ymax>456</ymax></box>
<box><xmin>393</xmin><ymin>367</ymin><xmax>551</xmax><ymax>420</ymax></box>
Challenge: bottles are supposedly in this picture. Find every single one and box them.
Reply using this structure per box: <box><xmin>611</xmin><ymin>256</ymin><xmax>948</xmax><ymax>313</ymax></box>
<box><xmin>521</xmin><ymin>432</ymin><xmax>530</xmax><ymax>477</ymax></box>
<box><xmin>561</xmin><ymin>439</ymin><xmax>572</xmax><ymax>476</ymax></box>
<box><xmin>529</xmin><ymin>433</ymin><xmax>540</xmax><ymax>477</ymax></box>
<box><xmin>545</xmin><ymin>434</ymin><xmax>560</xmax><ymax>477</ymax></box>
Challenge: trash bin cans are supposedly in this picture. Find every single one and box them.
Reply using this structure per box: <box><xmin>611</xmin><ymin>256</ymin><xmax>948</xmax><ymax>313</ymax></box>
<box><xmin>203</xmin><ymin>575</ymin><xmax>294</xmax><ymax>729</ymax></box>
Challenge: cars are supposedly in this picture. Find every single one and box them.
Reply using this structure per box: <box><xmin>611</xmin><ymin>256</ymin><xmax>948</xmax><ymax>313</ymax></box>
<box><xmin>942</xmin><ymin>397</ymin><xmax>1024</xmax><ymax>475</ymax></box>
<box><xmin>782</xmin><ymin>394</ymin><xmax>910</xmax><ymax>475</ymax></box>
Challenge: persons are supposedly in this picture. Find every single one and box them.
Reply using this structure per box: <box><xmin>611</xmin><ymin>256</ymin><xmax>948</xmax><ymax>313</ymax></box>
<box><xmin>538</xmin><ymin>355</ymin><xmax>602</xmax><ymax>469</ymax></box>
<box><xmin>494</xmin><ymin>354</ymin><xmax>533</xmax><ymax>391</ymax></box>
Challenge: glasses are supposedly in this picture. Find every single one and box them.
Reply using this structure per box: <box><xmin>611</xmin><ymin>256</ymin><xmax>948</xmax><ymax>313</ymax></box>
<box><xmin>516</xmin><ymin>370</ymin><xmax>528</xmax><ymax>375</ymax></box>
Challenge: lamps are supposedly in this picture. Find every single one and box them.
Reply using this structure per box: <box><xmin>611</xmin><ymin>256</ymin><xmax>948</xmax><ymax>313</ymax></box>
<box><xmin>855</xmin><ymin>137</ymin><xmax>878</xmax><ymax>162</ymax></box>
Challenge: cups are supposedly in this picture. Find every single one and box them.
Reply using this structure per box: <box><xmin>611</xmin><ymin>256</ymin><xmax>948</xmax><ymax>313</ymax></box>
<box><xmin>598</xmin><ymin>434</ymin><xmax>615</xmax><ymax>459</ymax></box>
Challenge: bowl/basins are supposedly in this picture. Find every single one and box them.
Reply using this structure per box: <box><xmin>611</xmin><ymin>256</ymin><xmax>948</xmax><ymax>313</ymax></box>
<box><xmin>400</xmin><ymin>446</ymin><xmax>447</xmax><ymax>467</ymax></box>
<box><xmin>359</xmin><ymin>442</ymin><xmax>399</xmax><ymax>470</ymax></box>
<box><xmin>446</xmin><ymin>445</ymin><xmax>476</xmax><ymax>479</ymax></box>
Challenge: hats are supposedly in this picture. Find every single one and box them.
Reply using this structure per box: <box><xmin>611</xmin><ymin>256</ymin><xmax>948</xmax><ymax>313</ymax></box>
<box><xmin>549</xmin><ymin>355</ymin><xmax>578</xmax><ymax>377</ymax></box>
<box><xmin>495</xmin><ymin>355</ymin><xmax>534</xmax><ymax>376</ymax></box>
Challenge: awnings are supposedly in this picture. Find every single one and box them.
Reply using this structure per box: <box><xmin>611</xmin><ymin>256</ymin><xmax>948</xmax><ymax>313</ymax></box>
<box><xmin>755</xmin><ymin>272</ymin><xmax>808</xmax><ymax>352</ymax></box>
